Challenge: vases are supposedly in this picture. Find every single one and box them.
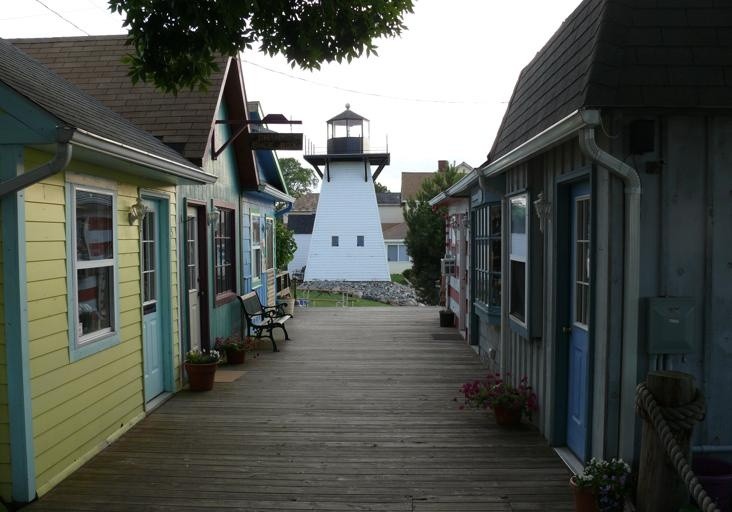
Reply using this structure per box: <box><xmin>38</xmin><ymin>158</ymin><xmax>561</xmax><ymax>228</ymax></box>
<box><xmin>225</xmin><ymin>348</ymin><xmax>245</xmax><ymax>363</ymax></box>
<box><xmin>494</xmin><ymin>405</ymin><xmax>522</xmax><ymax>425</ymax></box>
<box><xmin>569</xmin><ymin>476</ymin><xmax>596</xmax><ymax>511</ymax></box>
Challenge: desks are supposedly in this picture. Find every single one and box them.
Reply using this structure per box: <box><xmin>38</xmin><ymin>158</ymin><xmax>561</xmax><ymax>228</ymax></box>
<box><xmin>298</xmin><ymin>288</ymin><xmax>348</xmax><ymax>311</ymax></box>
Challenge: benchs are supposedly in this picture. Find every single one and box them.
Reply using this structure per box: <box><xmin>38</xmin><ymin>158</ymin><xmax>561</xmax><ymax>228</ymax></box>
<box><xmin>239</xmin><ymin>290</ymin><xmax>294</xmax><ymax>352</ymax></box>
<box><xmin>298</xmin><ymin>299</ymin><xmax>355</xmax><ymax>308</ymax></box>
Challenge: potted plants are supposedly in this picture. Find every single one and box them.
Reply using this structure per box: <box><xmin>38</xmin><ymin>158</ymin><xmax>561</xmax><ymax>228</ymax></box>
<box><xmin>184</xmin><ymin>347</ymin><xmax>221</xmax><ymax>391</ymax></box>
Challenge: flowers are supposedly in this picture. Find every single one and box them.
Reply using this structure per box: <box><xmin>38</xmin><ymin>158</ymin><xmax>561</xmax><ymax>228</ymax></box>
<box><xmin>455</xmin><ymin>368</ymin><xmax>538</xmax><ymax>423</ymax></box>
<box><xmin>575</xmin><ymin>457</ymin><xmax>632</xmax><ymax>511</ymax></box>
<box><xmin>216</xmin><ymin>330</ymin><xmax>263</xmax><ymax>367</ymax></box>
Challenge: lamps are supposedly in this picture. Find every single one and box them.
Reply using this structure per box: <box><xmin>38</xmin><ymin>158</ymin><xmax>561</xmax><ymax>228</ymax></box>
<box><xmin>128</xmin><ymin>198</ymin><xmax>148</xmax><ymax>226</ymax></box>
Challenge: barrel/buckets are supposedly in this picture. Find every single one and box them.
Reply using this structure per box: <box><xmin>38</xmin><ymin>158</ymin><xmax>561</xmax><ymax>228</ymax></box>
<box><xmin>275</xmin><ymin>298</ymin><xmax>295</xmax><ymax>316</ymax></box>
